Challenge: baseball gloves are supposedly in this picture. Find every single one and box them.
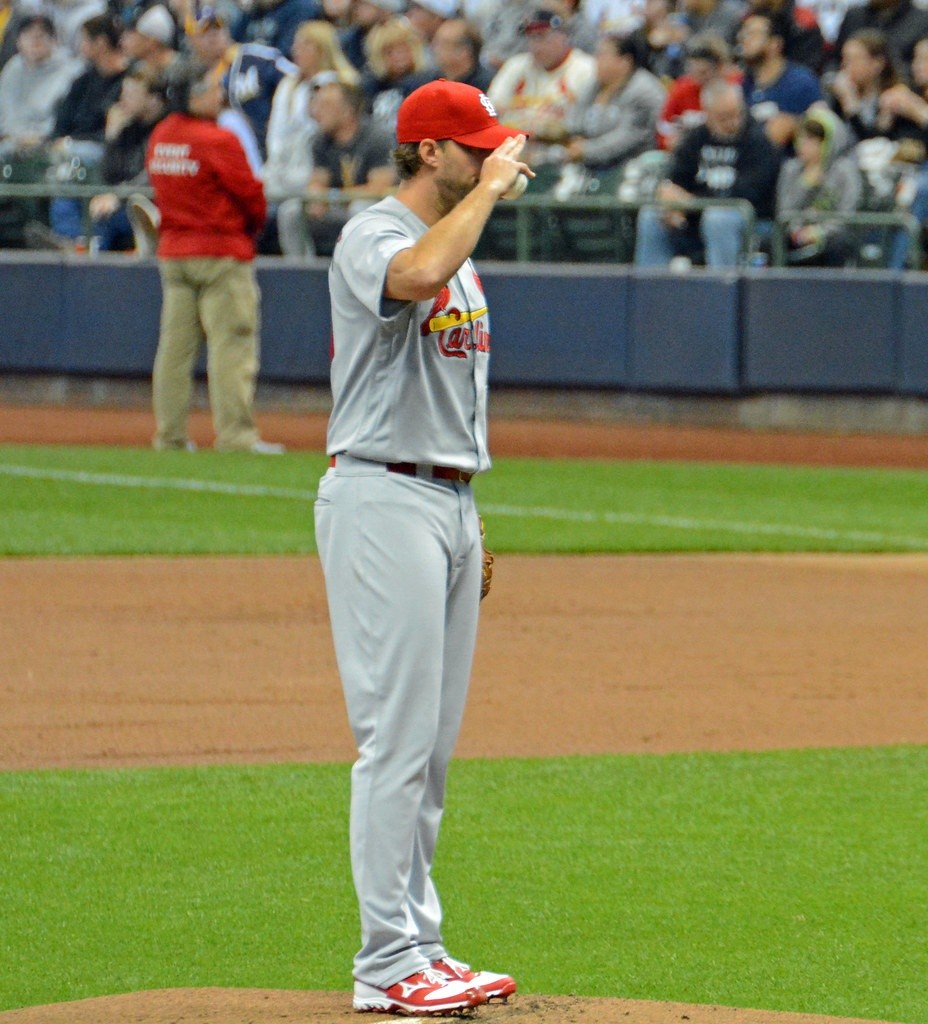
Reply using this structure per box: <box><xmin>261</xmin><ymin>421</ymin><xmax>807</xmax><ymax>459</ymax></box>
<box><xmin>476</xmin><ymin>518</ymin><xmax>495</xmax><ymax>603</ymax></box>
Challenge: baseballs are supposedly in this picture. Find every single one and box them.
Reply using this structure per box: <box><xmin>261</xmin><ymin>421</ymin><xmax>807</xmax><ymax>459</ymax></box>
<box><xmin>501</xmin><ymin>173</ymin><xmax>529</xmax><ymax>201</ymax></box>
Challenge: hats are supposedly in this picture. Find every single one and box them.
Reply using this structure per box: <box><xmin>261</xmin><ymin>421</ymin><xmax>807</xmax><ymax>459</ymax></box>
<box><xmin>515</xmin><ymin>9</ymin><xmax>565</xmax><ymax>36</ymax></box>
<box><xmin>138</xmin><ymin>5</ymin><xmax>178</xmax><ymax>48</ymax></box>
<box><xmin>392</xmin><ymin>78</ymin><xmax>534</xmax><ymax>154</ymax></box>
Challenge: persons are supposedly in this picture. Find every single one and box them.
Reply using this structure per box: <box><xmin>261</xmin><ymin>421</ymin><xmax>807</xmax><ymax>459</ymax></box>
<box><xmin>146</xmin><ymin>63</ymin><xmax>283</xmax><ymax>453</ymax></box>
<box><xmin>0</xmin><ymin>0</ymin><xmax>928</xmax><ymax>272</ymax></box>
<box><xmin>313</xmin><ymin>78</ymin><xmax>535</xmax><ymax>1018</ymax></box>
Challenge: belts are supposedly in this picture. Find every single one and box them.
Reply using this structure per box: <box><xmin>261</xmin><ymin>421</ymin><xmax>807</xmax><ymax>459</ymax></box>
<box><xmin>326</xmin><ymin>453</ymin><xmax>475</xmax><ymax>488</ymax></box>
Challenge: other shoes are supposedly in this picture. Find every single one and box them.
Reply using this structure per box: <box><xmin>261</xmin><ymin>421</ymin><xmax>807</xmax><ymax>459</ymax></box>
<box><xmin>220</xmin><ymin>438</ymin><xmax>291</xmax><ymax>458</ymax></box>
<box><xmin>153</xmin><ymin>436</ymin><xmax>202</xmax><ymax>453</ymax></box>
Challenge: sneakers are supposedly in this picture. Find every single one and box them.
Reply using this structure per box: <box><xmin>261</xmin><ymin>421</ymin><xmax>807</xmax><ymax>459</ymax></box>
<box><xmin>432</xmin><ymin>953</ymin><xmax>520</xmax><ymax>1000</ymax></box>
<box><xmin>350</xmin><ymin>965</ymin><xmax>490</xmax><ymax>1018</ymax></box>
